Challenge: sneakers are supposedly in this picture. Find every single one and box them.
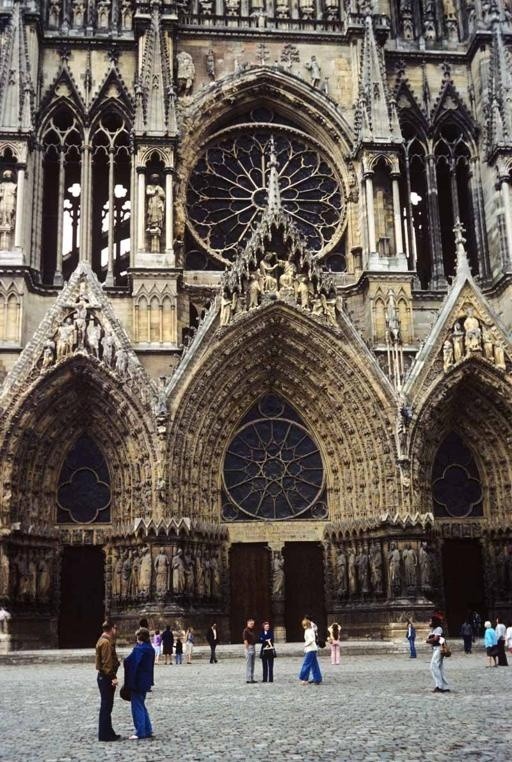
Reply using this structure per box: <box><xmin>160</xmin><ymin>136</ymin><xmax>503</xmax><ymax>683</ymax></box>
<box><xmin>129</xmin><ymin>735</ymin><xmax>139</xmax><ymax>739</ymax></box>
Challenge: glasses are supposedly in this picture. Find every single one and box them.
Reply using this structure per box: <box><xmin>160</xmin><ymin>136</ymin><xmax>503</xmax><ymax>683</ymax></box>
<box><xmin>113</xmin><ymin>626</ymin><xmax>118</xmax><ymax>630</ymax></box>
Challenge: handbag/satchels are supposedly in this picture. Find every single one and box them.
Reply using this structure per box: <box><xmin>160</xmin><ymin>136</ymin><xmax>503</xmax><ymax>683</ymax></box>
<box><xmin>120</xmin><ymin>683</ymin><xmax>131</xmax><ymax>700</ymax></box>
<box><xmin>263</xmin><ymin>639</ymin><xmax>277</xmax><ymax>658</ymax></box>
<box><xmin>441</xmin><ymin>636</ymin><xmax>452</xmax><ymax>656</ymax></box>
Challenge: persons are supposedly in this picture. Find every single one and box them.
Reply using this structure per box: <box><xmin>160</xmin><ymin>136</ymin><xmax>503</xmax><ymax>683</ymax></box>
<box><xmin>494</xmin><ymin>617</ymin><xmax>508</xmax><ymax>666</ymax></box>
<box><xmin>96</xmin><ymin>621</ymin><xmax>120</xmax><ymax>741</ymax></box>
<box><xmin>206</xmin><ymin>622</ymin><xmax>218</xmax><ymax>663</ymax></box>
<box><xmin>471</xmin><ymin>613</ymin><xmax>477</xmax><ymax>642</ymax></box>
<box><xmin>406</xmin><ymin>617</ymin><xmax>416</xmax><ymax>658</ymax></box>
<box><xmin>426</xmin><ymin>616</ymin><xmax>450</xmax><ymax>693</ymax></box>
<box><xmin>507</xmin><ymin>622</ymin><xmax>512</xmax><ymax>654</ymax></box>
<box><xmin>161</xmin><ymin>626</ymin><xmax>173</xmax><ymax>665</ymax></box>
<box><xmin>172</xmin><ymin>639</ymin><xmax>183</xmax><ymax>664</ymax></box>
<box><xmin>328</xmin><ymin>623</ymin><xmax>341</xmax><ymax>665</ymax></box>
<box><xmin>461</xmin><ymin>618</ymin><xmax>473</xmax><ymax>654</ymax></box>
<box><xmin>135</xmin><ymin>619</ymin><xmax>155</xmax><ymax>686</ymax></box>
<box><xmin>474</xmin><ymin>609</ymin><xmax>481</xmax><ymax>640</ymax></box>
<box><xmin>436</xmin><ymin>612</ymin><xmax>452</xmax><ymax>657</ymax></box>
<box><xmin>125</xmin><ymin>629</ymin><xmax>156</xmax><ymax>741</ymax></box>
<box><xmin>304</xmin><ymin>615</ymin><xmax>317</xmax><ymax>683</ymax></box>
<box><xmin>154</xmin><ymin>631</ymin><xmax>161</xmax><ymax>665</ymax></box>
<box><xmin>185</xmin><ymin>627</ymin><xmax>195</xmax><ymax>664</ymax></box>
<box><xmin>299</xmin><ymin>620</ymin><xmax>322</xmax><ymax>685</ymax></box>
<box><xmin>243</xmin><ymin>619</ymin><xmax>257</xmax><ymax>683</ymax></box>
<box><xmin>258</xmin><ymin>622</ymin><xmax>277</xmax><ymax>682</ymax></box>
<box><xmin>484</xmin><ymin>621</ymin><xmax>498</xmax><ymax>668</ymax></box>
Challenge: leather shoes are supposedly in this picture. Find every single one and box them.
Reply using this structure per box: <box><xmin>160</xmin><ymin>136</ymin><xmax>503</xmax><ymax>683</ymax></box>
<box><xmin>431</xmin><ymin>687</ymin><xmax>440</xmax><ymax>693</ymax></box>
<box><xmin>113</xmin><ymin>734</ymin><xmax>121</xmax><ymax>739</ymax></box>
<box><xmin>247</xmin><ymin>680</ymin><xmax>257</xmax><ymax>683</ymax></box>
<box><xmin>100</xmin><ymin>736</ymin><xmax>118</xmax><ymax>741</ymax></box>
<box><xmin>441</xmin><ymin>689</ymin><xmax>449</xmax><ymax>692</ymax></box>
